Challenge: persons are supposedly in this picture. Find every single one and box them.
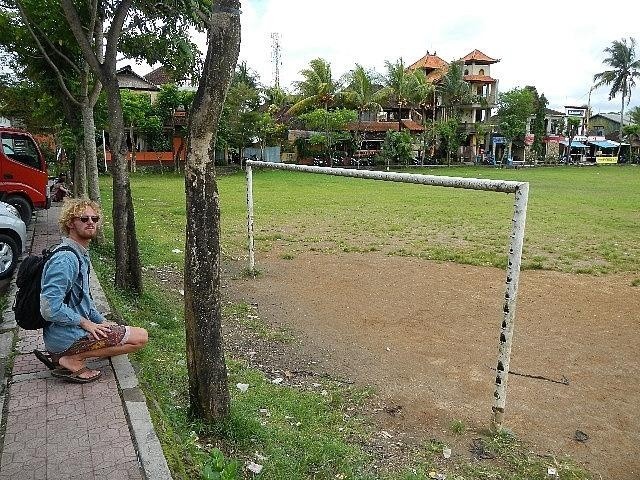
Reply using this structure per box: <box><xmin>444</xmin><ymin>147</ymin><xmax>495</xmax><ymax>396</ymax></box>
<box><xmin>50</xmin><ymin>174</ymin><xmax>69</xmax><ymax>203</ymax></box>
<box><xmin>39</xmin><ymin>198</ymin><xmax>149</xmax><ymax>383</ymax></box>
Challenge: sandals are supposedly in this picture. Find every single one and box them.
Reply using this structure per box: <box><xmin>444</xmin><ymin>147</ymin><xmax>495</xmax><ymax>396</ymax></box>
<box><xmin>33</xmin><ymin>348</ymin><xmax>102</xmax><ymax>383</ymax></box>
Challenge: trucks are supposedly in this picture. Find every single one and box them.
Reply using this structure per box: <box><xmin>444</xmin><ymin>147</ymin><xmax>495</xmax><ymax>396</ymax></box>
<box><xmin>0</xmin><ymin>126</ymin><xmax>52</xmax><ymax>228</ymax></box>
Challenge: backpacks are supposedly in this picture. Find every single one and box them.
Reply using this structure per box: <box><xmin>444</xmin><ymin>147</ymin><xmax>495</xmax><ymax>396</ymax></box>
<box><xmin>15</xmin><ymin>245</ymin><xmax>82</xmax><ymax>330</ymax></box>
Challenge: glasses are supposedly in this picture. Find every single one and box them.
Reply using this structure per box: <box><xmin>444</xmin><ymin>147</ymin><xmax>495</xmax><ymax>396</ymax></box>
<box><xmin>73</xmin><ymin>216</ymin><xmax>100</xmax><ymax>223</ymax></box>
